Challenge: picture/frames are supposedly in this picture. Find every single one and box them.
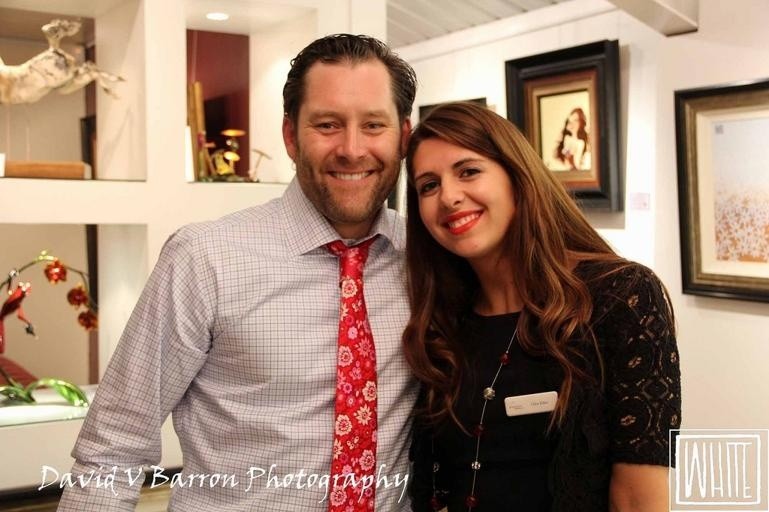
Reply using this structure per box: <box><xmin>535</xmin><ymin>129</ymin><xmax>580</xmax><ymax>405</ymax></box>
<box><xmin>674</xmin><ymin>81</ymin><xmax>768</xmax><ymax>303</ymax></box>
<box><xmin>506</xmin><ymin>40</ymin><xmax>620</xmax><ymax>211</ymax></box>
<box><xmin>420</xmin><ymin>97</ymin><xmax>486</xmax><ymax>118</ymax></box>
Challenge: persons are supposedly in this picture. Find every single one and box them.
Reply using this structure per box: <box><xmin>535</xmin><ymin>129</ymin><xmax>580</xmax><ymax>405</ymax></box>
<box><xmin>404</xmin><ymin>98</ymin><xmax>684</xmax><ymax>512</ymax></box>
<box><xmin>550</xmin><ymin>107</ymin><xmax>591</xmax><ymax>172</ymax></box>
<box><xmin>51</xmin><ymin>29</ymin><xmax>425</xmax><ymax>511</ymax></box>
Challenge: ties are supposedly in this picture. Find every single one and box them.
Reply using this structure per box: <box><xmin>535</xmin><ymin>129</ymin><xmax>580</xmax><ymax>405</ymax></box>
<box><xmin>324</xmin><ymin>241</ymin><xmax>380</xmax><ymax>509</ymax></box>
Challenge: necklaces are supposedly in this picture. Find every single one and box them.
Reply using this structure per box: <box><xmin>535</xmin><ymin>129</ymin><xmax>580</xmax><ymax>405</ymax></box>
<box><xmin>421</xmin><ymin>315</ymin><xmax>524</xmax><ymax>511</ymax></box>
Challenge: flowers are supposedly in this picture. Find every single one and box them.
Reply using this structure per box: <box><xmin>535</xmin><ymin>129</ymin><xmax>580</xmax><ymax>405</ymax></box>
<box><xmin>1</xmin><ymin>251</ymin><xmax>99</xmax><ymax>406</ymax></box>
